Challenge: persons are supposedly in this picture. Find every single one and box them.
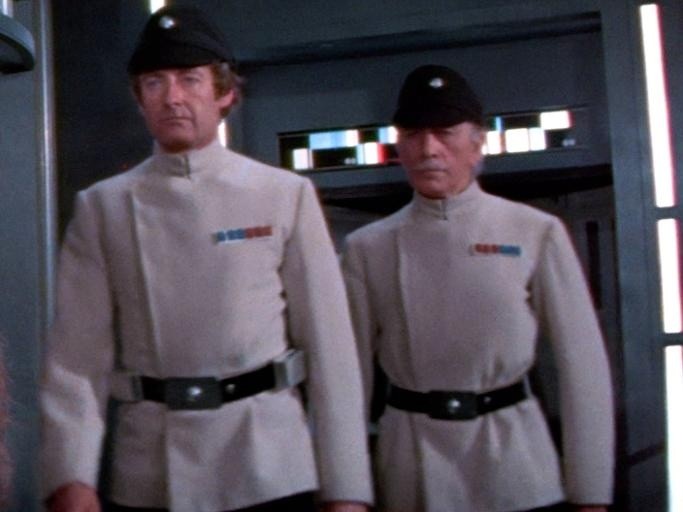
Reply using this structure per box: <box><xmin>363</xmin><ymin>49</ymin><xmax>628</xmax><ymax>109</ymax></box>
<box><xmin>339</xmin><ymin>64</ymin><xmax>618</xmax><ymax>509</ymax></box>
<box><xmin>39</xmin><ymin>6</ymin><xmax>377</xmax><ymax>510</ymax></box>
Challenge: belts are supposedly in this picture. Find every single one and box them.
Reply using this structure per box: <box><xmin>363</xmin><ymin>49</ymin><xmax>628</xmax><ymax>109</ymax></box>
<box><xmin>388</xmin><ymin>380</ymin><xmax>525</xmax><ymax>420</ymax></box>
<box><xmin>139</xmin><ymin>362</ymin><xmax>276</xmax><ymax>410</ymax></box>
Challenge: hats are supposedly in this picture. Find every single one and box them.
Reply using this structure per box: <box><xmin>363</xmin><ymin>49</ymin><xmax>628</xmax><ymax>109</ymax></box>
<box><xmin>392</xmin><ymin>65</ymin><xmax>483</xmax><ymax>128</ymax></box>
<box><xmin>125</xmin><ymin>6</ymin><xmax>216</xmax><ymax>75</ymax></box>
